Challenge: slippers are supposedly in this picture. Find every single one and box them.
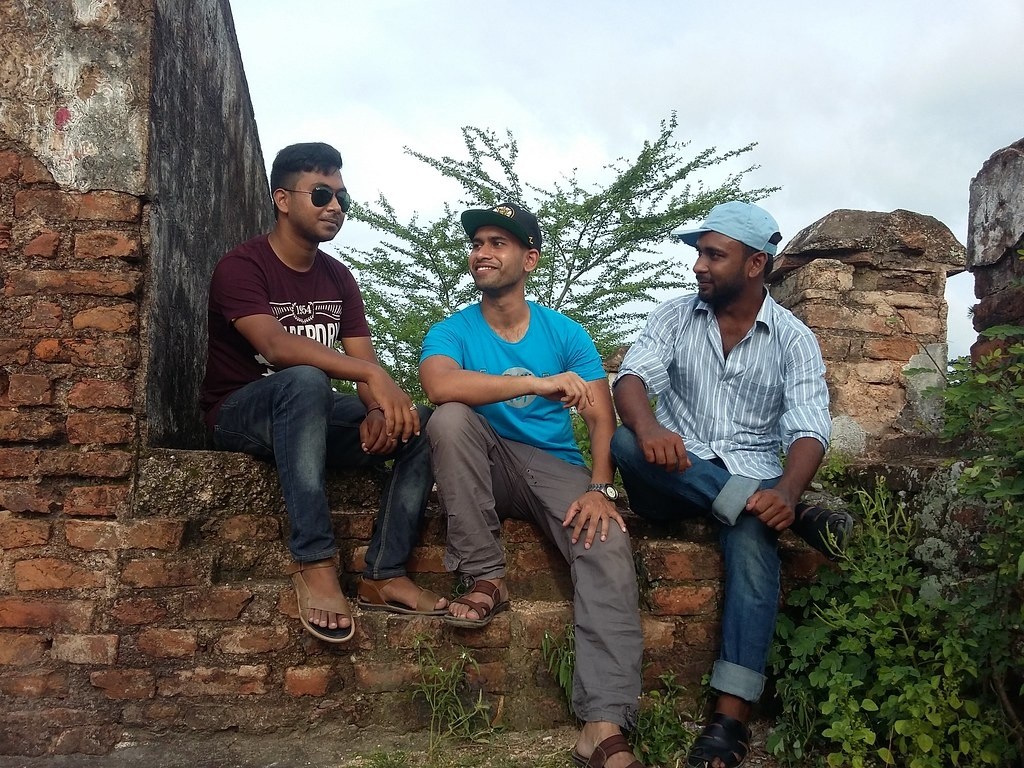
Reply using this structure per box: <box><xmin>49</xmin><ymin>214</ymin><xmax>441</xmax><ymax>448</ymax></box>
<box><xmin>444</xmin><ymin>580</ymin><xmax>511</xmax><ymax>628</ymax></box>
<box><xmin>572</xmin><ymin>734</ymin><xmax>646</xmax><ymax>768</ymax></box>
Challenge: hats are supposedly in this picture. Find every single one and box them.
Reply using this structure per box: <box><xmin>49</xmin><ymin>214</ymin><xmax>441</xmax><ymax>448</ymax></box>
<box><xmin>461</xmin><ymin>203</ymin><xmax>542</xmax><ymax>258</ymax></box>
<box><xmin>672</xmin><ymin>201</ymin><xmax>782</xmax><ymax>256</ymax></box>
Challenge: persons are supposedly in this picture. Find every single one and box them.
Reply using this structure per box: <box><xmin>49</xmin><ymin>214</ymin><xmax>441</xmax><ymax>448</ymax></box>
<box><xmin>200</xmin><ymin>141</ymin><xmax>452</xmax><ymax>643</ymax></box>
<box><xmin>609</xmin><ymin>198</ymin><xmax>864</xmax><ymax>768</ymax></box>
<box><xmin>420</xmin><ymin>200</ymin><xmax>657</xmax><ymax>767</ymax></box>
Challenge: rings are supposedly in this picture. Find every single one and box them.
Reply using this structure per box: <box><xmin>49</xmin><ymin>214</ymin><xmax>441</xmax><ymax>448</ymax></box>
<box><xmin>409</xmin><ymin>403</ymin><xmax>418</xmax><ymax>411</ymax></box>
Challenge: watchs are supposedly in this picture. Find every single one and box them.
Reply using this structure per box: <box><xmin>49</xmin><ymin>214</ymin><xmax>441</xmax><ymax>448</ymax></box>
<box><xmin>584</xmin><ymin>482</ymin><xmax>620</xmax><ymax>501</ymax></box>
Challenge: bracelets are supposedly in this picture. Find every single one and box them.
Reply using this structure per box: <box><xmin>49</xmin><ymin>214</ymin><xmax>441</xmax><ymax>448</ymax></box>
<box><xmin>366</xmin><ymin>405</ymin><xmax>385</xmax><ymax>417</ymax></box>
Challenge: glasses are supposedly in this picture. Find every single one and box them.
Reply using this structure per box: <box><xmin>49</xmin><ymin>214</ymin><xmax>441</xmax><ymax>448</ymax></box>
<box><xmin>281</xmin><ymin>185</ymin><xmax>352</xmax><ymax>214</ymax></box>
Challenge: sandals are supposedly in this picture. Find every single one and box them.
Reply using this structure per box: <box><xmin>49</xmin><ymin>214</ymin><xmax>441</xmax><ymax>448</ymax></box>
<box><xmin>286</xmin><ymin>558</ymin><xmax>355</xmax><ymax>642</ymax></box>
<box><xmin>787</xmin><ymin>504</ymin><xmax>854</xmax><ymax>561</ymax></box>
<box><xmin>687</xmin><ymin>712</ymin><xmax>751</xmax><ymax>768</ymax></box>
<box><xmin>357</xmin><ymin>574</ymin><xmax>451</xmax><ymax>615</ymax></box>
